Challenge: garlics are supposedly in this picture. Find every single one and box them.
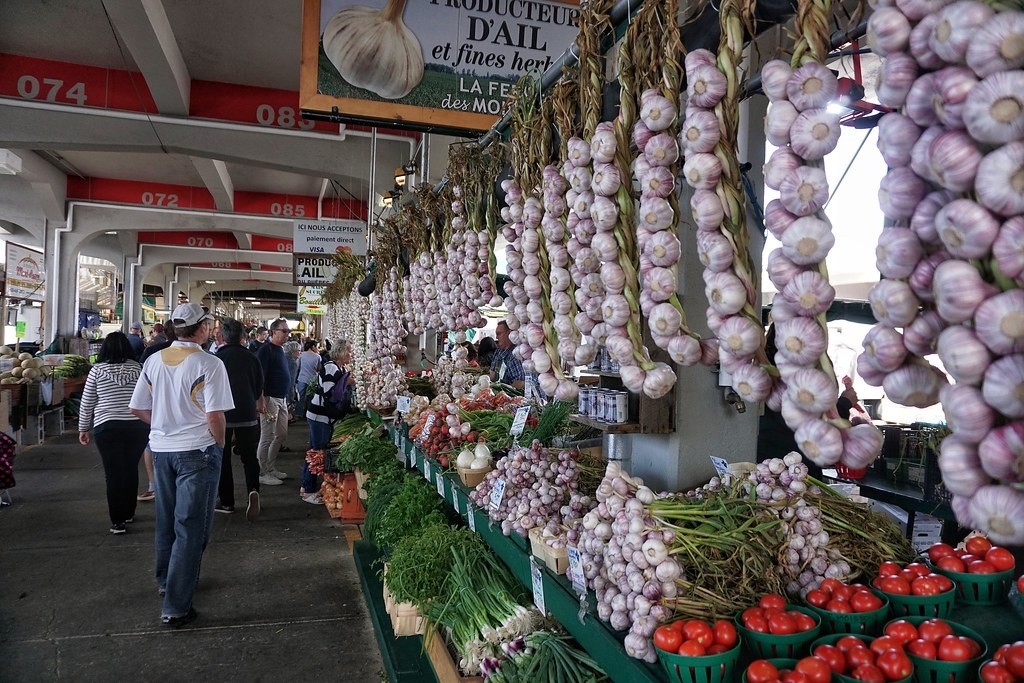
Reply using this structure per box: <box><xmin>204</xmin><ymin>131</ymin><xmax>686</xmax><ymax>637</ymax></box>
<box><xmin>466</xmin><ymin>438</ymin><xmax>852</xmax><ymax>664</ymax></box>
<box><xmin>322</xmin><ymin>0</ymin><xmax>425</xmax><ymax>99</ymax></box>
<box><xmin>326</xmin><ymin>184</ymin><xmax>503</xmax><ymax>409</ymax></box>
<box><xmin>496</xmin><ymin>0</ymin><xmax>1024</xmax><ymax>544</ymax></box>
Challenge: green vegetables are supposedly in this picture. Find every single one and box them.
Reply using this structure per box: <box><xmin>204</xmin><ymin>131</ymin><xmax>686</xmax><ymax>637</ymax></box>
<box><xmin>336</xmin><ymin>421</ymin><xmax>485</xmax><ymax>604</ymax></box>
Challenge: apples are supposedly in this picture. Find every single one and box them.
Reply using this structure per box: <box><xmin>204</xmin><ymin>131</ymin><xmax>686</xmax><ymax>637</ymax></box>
<box><xmin>321</xmin><ymin>473</ymin><xmax>352</xmax><ymax>510</ymax></box>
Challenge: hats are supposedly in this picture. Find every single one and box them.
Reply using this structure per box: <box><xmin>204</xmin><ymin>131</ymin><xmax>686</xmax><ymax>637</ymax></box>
<box><xmin>132</xmin><ymin>322</ymin><xmax>143</xmax><ymax>329</ymax></box>
<box><xmin>152</xmin><ymin>324</ymin><xmax>164</xmax><ymax>334</ymax></box>
<box><xmin>171</xmin><ymin>303</ymin><xmax>213</xmax><ymax>328</ymax></box>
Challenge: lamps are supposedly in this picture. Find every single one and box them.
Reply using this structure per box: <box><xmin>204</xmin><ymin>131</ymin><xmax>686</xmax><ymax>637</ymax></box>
<box><xmin>384</xmin><ymin>159</ymin><xmax>417</xmax><ymax>208</ymax></box>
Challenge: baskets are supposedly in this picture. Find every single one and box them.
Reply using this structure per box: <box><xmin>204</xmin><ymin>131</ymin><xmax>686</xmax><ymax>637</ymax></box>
<box><xmin>652</xmin><ymin>555</ymin><xmax>1024</xmax><ymax>683</ymax></box>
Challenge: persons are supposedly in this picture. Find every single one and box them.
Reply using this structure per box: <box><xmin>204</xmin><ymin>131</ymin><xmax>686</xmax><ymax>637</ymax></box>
<box><xmin>129</xmin><ymin>303</ymin><xmax>235</xmax><ymax>628</ymax></box>
<box><xmin>128</xmin><ymin>308</ymin><xmax>356</xmax><ymax>519</ymax></box>
<box><xmin>448</xmin><ymin>320</ymin><xmax>548</xmax><ymax>402</ymax></box>
<box><xmin>78</xmin><ymin>333</ymin><xmax>152</xmax><ymax>533</ymax></box>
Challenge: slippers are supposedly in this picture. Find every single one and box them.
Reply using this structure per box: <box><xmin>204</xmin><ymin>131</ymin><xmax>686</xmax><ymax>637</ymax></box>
<box><xmin>301</xmin><ymin>492</ymin><xmax>325</xmax><ymax>505</ymax></box>
<box><xmin>300</xmin><ymin>487</ymin><xmax>305</xmax><ymax>496</ymax></box>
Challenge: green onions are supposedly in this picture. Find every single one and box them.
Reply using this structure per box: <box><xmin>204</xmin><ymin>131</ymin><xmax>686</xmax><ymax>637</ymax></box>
<box><xmin>413</xmin><ymin>542</ymin><xmax>612</xmax><ymax>683</ymax></box>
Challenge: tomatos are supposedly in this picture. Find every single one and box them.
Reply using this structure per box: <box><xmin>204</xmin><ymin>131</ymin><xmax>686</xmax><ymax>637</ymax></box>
<box><xmin>650</xmin><ymin>535</ymin><xmax>1024</xmax><ymax>683</ymax></box>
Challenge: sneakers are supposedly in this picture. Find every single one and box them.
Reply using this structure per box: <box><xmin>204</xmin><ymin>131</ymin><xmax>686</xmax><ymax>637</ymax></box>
<box><xmin>214</xmin><ymin>498</ymin><xmax>235</xmax><ymax>514</ymax></box>
<box><xmin>246</xmin><ymin>491</ymin><xmax>260</xmax><ymax>522</ymax></box>
<box><xmin>268</xmin><ymin>468</ymin><xmax>287</xmax><ymax>479</ymax></box>
<box><xmin>126</xmin><ymin>514</ymin><xmax>136</xmax><ymax>522</ymax></box>
<box><xmin>110</xmin><ymin>522</ymin><xmax>126</xmax><ymax>533</ymax></box>
<box><xmin>137</xmin><ymin>488</ymin><xmax>155</xmax><ymax>500</ymax></box>
<box><xmin>258</xmin><ymin>472</ymin><xmax>283</xmax><ymax>485</ymax></box>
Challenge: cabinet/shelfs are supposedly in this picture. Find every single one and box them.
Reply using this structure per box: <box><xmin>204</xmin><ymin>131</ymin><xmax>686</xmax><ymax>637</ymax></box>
<box><xmin>569</xmin><ymin>298</ymin><xmax>676</xmax><ymax>435</ymax></box>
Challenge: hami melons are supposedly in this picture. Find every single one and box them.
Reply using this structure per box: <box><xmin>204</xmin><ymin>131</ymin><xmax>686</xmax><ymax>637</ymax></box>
<box><xmin>0</xmin><ymin>346</ymin><xmax>51</xmax><ymax>383</ymax></box>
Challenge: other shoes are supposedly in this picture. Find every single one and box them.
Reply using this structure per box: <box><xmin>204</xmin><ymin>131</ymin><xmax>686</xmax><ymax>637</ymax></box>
<box><xmin>158</xmin><ymin>606</ymin><xmax>197</xmax><ymax>628</ymax></box>
<box><xmin>158</xmin><ymin>583</ymin><xmax>166</xmax><ymax>596</ymax></box>
<box><xmin>279</xmin><ymin>444</ymin><xmax>290</xmax><ymax>452</ymax></box>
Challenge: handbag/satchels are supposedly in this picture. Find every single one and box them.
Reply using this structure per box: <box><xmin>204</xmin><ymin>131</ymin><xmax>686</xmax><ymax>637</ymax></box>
<box><xmin>325</xmin><ymin>390</ymin><xmax>350</xmax><ymax>420</ymax></box>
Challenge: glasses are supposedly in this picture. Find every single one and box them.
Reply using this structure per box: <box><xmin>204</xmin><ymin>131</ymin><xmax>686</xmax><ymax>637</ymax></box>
<box><xmin>197</xmin><ymin>307</ymin><xmax>210</xmax><ymax>323</ymax></box>
<box><xmin>273</xmin><ymin>329</ymin><xmax>291</xmax><ymax>333</ymax></box>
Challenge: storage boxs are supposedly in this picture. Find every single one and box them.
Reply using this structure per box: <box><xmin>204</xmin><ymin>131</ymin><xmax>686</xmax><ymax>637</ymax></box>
<box><xmin>323</xmin><ymin>408</ymin><xmax>1024</xmax><ymax>683</ymax></box>
<box><xmin>0</xmin><ymin>338</ymin><xmax>104</xmax><ymax>432</ymax></box>
